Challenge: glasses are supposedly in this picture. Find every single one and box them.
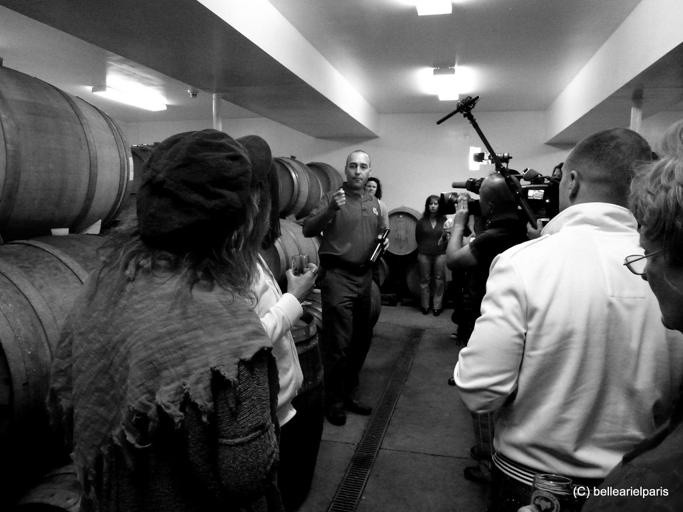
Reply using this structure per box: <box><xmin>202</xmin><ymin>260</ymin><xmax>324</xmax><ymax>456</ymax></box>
<box><xmin>624</xmin><ymin>249</ymin><xmax>664</xmax><ymax>275</ymax></box>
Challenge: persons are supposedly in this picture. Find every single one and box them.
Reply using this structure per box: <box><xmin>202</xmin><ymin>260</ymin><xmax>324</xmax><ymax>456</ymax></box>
<box><xmin>413</xmin><ymin>194</ymin><xmax>450</xmax><ymax>316</ymax></box>
<box><xmin>44</xmin><ymin>131</ymin><xmax>391</xmax><ymax>512</ymax></box>
<box><xmin>441</xmin><ymin>123</ymin><xmax>682</xmax><ymax>511</ymax></box>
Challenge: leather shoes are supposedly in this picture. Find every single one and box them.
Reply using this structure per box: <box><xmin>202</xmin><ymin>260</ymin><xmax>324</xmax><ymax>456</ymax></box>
<box><xmin>325</xmin><ymin>400</ymin><xmax>371</xmax><ymax>426</ymax></box>
<box><xmin>464</xmin><ymin>446</ymin><xmax>490</xmax><ymax>482</ymax></box>
<box><xmin>423</xmin><ymin>308</ymin><xmax>441</xmax><ymax>316</ymax></box>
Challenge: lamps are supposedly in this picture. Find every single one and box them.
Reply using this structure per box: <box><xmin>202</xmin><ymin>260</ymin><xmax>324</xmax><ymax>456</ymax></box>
<box><xmin>433</xmin><ymin>65</ymin><xmax>459</xmax><ymax>101</ymax></box>
<box><xmin>91</xmin><ymin>85</ymin><xmax>166</xmax><ymax>111</ymax></box>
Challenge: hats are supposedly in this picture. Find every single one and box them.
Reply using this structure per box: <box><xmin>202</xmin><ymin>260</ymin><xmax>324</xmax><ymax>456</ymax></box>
<box><xmin>136</xmin><ymin>129</ymin><xmax>272</xmax><ymax>255</ymax></box>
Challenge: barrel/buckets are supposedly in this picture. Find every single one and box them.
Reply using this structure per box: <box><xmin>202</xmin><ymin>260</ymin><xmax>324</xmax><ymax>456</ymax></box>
<box><xmin>301</xmin><ymin>290</ymin><xmax>322</xmax><ymax>331</ymax></box>
<box><xmin>291</xmin><ymin>312</ymin><xmax>326</xmax><ymax>457</ymax></box>
<box><xmin>306</xmin><ymin>163</ymin><xmax>343</xmax><ymax>191</ymax></box>
<box><xmin>368</xmin><ymin>280</ymin><xmax>382</xmax><ymax>326</ymax></box>
<box><xmin>386</xmin><ymin>206</ymin><xmax>423</xmax><ymax>256</ymax></box>
<box><xmin>265</xmin><ymin>222</ymin><xmax>326</xmax><ymax>289</ymax></box>
<box><xmin>0</xmin><ymin>234</ymin><xmax>119</xmax><ymax>423</ymax></box>
<box><xmin>407</xmin><ymin>258</ymin><xmax>448</xmax><ymax>297</ymax></box>
<box><xmin>0</xmin><ymin>66</ymin><xmax>129</xmax><ymax>234</ymax></box>
<box><xmin>273</xmin><ymin>156</ymin><xmax>322</xmax><ymax>217</ymax></box>
<box><xmin>379</xmin><ymin>256</ymin><xmax>387</xmax><ymax>285</ymax></box>
